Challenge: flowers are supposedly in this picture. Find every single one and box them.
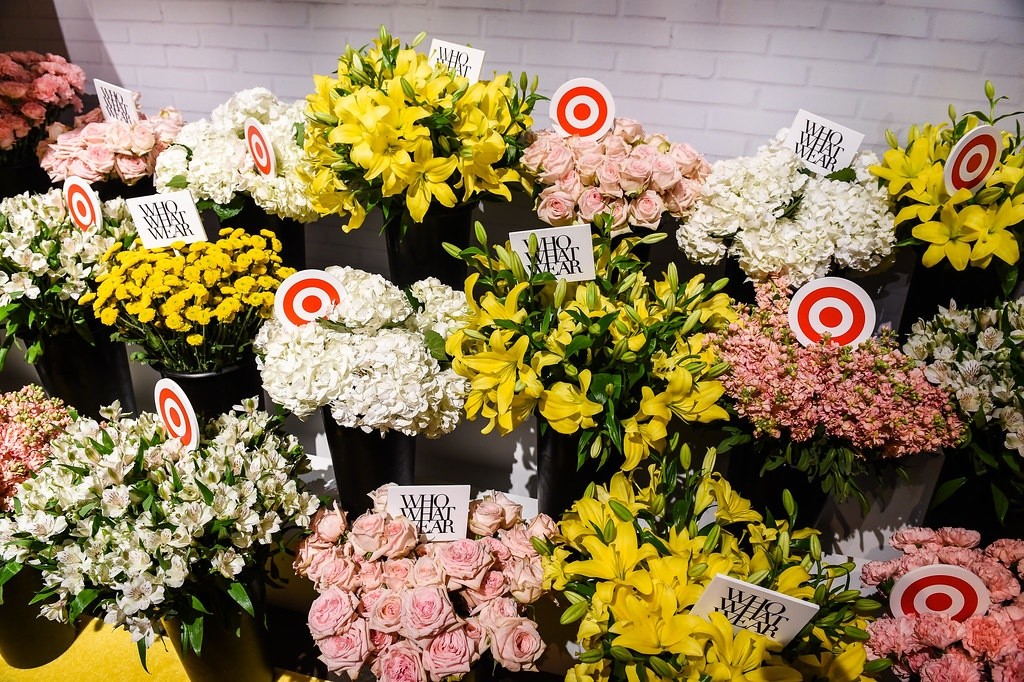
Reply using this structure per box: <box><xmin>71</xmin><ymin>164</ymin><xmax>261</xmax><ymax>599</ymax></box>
<box><xmin>0</xmin><ymin>37</ymin><xmax>1024</xmax><ymax>682</ymax></box>
